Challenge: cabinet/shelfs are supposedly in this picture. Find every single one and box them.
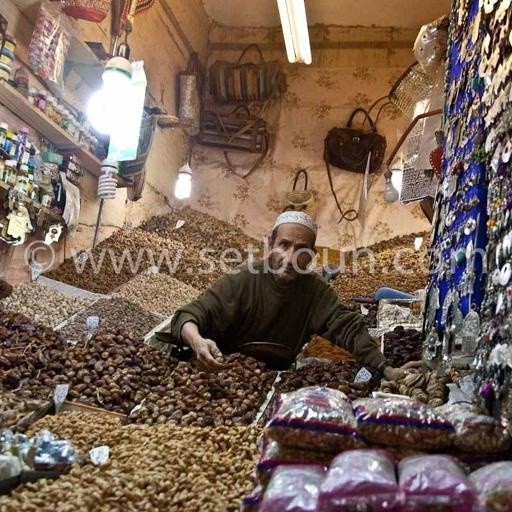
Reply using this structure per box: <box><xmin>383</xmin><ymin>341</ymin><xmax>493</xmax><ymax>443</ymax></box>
<box><xmin>1</xmin><ymin>77</ymin><xmax>124</xmax><ymax>229</ymax></box>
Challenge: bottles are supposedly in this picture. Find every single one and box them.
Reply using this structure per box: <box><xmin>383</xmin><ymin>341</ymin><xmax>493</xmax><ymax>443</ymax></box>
<box><xmin>10</xmin><ymin>65</ymin><xmax>107</xmax><ymax>162</ymax></box>
<box><xmin>0</xmin><ymin>122</ymin><xmax>63</xmax><ymax>208</ymax></box>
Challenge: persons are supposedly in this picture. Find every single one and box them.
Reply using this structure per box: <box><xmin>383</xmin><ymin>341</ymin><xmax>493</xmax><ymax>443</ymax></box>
<box><xmin>171</xmin><ymin>210</ymin><xmax>425</xmax><ymax>384</ymax></box>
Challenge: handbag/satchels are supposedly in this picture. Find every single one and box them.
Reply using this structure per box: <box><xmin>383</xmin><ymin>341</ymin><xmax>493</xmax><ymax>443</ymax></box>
<box><xmin>282</xmin><ymin>191</ymin><xmax>316</xmax><ymax>220</ymax></box>
<box><xmin>323</xmin><ymin>126</ymin><xmax>386</xmax><ymax>173</ymax></box>
<box><xmin>208</xmin><ymin>60</ymin><xmax>286</xmax><ymax>106</ymax></box>
<box><xmin>389</xmin><ymin>15</ymin><xmax>447</xmax><ymax>205</ymax></box>
<box><xmin>178</xmin><ymin>70</ymin><xmax>201</xmax><ymax>136</ymax></box>
<box><xmin>196</xmin><ymin>110</ymin><xmax>266</xmax><ymax>154</ymax></box>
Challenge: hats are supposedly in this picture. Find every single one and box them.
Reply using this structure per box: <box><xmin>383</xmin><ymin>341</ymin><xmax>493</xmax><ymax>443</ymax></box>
<box><xmin>272</xmin><ymin>211</ymin><xmax>317</xmax><ymax>240</ymax></box>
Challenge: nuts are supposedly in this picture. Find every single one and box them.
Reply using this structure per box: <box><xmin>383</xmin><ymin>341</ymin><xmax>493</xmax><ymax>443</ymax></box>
<box><xmin>0</xmin><ymin>204</ymin><xmax>512</xmax><ymax>512</ymax></box>
